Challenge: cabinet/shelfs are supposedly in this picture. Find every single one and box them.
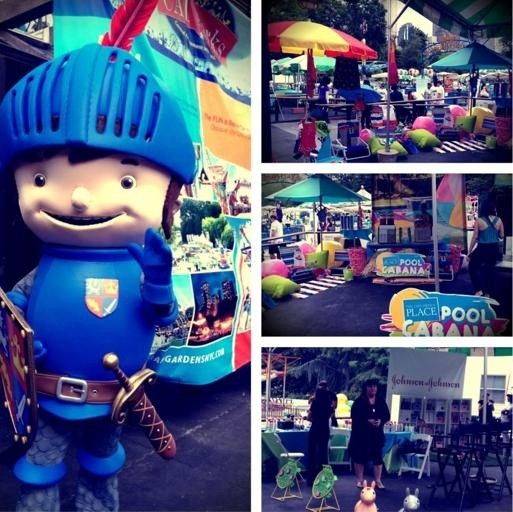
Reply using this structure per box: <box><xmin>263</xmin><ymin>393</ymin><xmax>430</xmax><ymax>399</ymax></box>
<box><xmin>423</xmin><ymin>397</ymin><xmax>449</xmax><ymax>446</ymax></box>
<box><xmin>388</xmin><ymin>392</ymin><xmax>424</xmax><ymax>436</ymax></box>
<box><xmin>448</xmin><ymin>397</ymin><xmax>473</xmax><ymax>428</ymax></box>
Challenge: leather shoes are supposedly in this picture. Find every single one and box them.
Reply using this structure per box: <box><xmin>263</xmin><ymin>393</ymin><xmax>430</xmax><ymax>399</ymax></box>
<box><xmin>375</xmin><ymin>479</ymin><xmax>387</xmax><ymax>491</ymax></box>
<box><xmin>356</xmin><ymin>480</ymin><xmax>364</xmax><ymax>488</ymax></box>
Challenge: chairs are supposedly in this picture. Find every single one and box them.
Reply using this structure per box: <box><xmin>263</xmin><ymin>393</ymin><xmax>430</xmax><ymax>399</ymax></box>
<box><xmin>261</xmin><ymin>417</ymin><xmax>512</xmax><ymax>512</ymax></box>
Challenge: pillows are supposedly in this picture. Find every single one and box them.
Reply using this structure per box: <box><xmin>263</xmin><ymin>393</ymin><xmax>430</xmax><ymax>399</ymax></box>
<box><xmin>261</xmin><ymin>272</ymin><xmax>300</xmax><ymax>300</ymax></box>
<box><xmin>262</xmin><ymin>258</ymin><xmax>288</xmax><ymax>277</ymax></box>
<box><xmin>345</xmin><ymin>245</ymin><xmax>369</xmax><ymax>275</ymax></box>
<box><xmin>356</xmin><ymin>103</ymin><xmax>500</xmax><ymax>153</ymax></box>
<box><xmin>306</xmin><ymin>248</ymin><xmax>327</xmax><ymax>268</ymax></box>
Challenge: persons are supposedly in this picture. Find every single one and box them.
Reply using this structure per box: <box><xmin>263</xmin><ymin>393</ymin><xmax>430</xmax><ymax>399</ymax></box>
<box><xmin>317</xmin><ymin>70</ymin><xmax>504</xmax><ymax>130</ymax></box>
<box><xmin>268</xmin><ymin>201</ymin><xmax>368</xmax><ymax>259</ymax></box>
<box><xmin>465</xmin><ymin>200</ymin><xmax>504</xmax><ymax>299</ymax></box>
<box><xmin>304</xmin><ymin>377</ymin><xmax>511</xmax><ymax>490</ymax></box>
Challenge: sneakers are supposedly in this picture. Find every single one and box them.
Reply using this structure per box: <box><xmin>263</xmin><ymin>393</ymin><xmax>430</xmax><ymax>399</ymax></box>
<box><xmin>474</xmin><ymin>290</ymin><xmax>483</xmax><ymax>296</ymax></box>
<box><xmin>484</xmin><ymin>293</ymin><xmax>491</xmax><ymax>298</ymax></box>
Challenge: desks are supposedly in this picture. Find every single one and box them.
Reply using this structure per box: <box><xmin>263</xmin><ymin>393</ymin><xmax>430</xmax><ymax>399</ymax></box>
<box><xmin>366</xmin><ymin>239</ymin><xmax>455</xmax><ymax>277</ymax></box>
<box><xmin>269</xmin><ymin>85</ymin><xmax>359</xmax><ymax>122</ymax></box>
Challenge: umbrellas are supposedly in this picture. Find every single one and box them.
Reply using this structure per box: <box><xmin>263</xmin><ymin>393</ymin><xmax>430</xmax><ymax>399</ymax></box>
<box><xmin>427</xmin><ymin>41</ymin><xmax>510</xmax><ymax>99</ymax></box>
<box><xmin>267</xmin><ymin>18</ymin><xmax>374</xmax><ymax>98</ymax></box>
<box><xmin>265</xmin><ymin>173</ymin><xmax>368</xmax><ymax>250</ymax></box>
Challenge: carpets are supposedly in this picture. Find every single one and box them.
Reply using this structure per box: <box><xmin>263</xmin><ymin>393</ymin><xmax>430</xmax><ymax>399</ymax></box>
<box><xmin>290</xmin><ymin>272</ymin><xmax>350</xmax><ymax>299</ymax></box>
